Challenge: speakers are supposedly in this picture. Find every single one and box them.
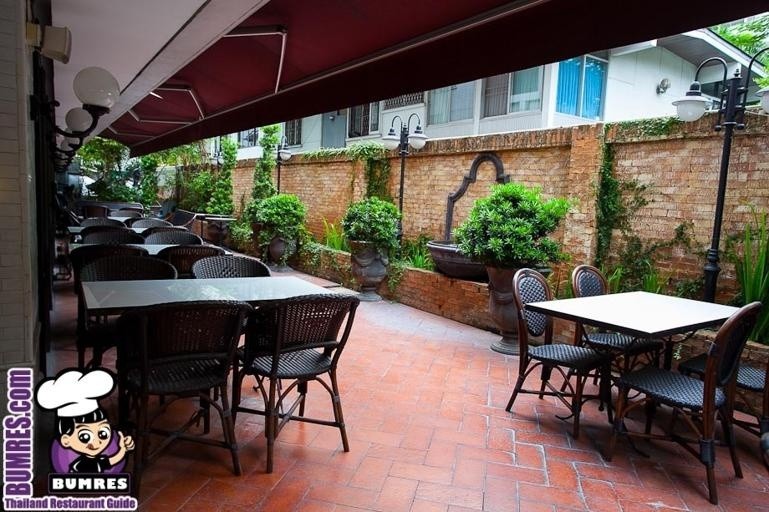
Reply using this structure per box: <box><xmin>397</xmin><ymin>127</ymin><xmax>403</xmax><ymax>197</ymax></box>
<box><xmin>43</xmin><ymin>26</ymin><xmax>72</xmax><ymax>64</ymax></box>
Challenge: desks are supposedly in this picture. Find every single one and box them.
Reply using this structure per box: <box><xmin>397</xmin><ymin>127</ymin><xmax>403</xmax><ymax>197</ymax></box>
<box><xmin>524</xmin><ymin>287</ymin><xmax>742</xmax><ymax>460</ymax></box>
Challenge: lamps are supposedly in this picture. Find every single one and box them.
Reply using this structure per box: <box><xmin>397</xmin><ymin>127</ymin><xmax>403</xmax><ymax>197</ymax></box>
<box><xmin>33</xmin><ymin>43</ymin><xmax>122</xmax><ymax>180</ymax></box>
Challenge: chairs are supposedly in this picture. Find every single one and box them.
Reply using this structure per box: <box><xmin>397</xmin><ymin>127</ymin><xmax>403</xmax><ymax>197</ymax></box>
<box><xmin>614</xmin><ymin>299</ymin><xmax>764</xmax><ymax>506</ymax></box>
<box><xmin>669</xmin><ymin>351</ymin><xmax>769</xmax><ymax>474</ymax></box>
<box><xmin>505</xmin><ymin>267</ymin><xmax>612</xmax><ymax>441</ymax></box>
<box><xmin>561</xmin><ymin>261</ymin><xmax>666</xmax><ymax>423</ymax></box>
<box><xmin>51</xmin><ymin>188</ymin><xmax>363</xmax><ymax>512</ymax></box>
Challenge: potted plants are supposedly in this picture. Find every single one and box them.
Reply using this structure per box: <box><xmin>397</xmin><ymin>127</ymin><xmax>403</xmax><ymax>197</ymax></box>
<box><xmin>449</xmin><ymin>181</ymin><xmax>584</xmax><ymax>358</ymax></box>
<box><xmin>204</xmin><ymin>137</ymin><xmax>239</xmax><ymax>239</ymax></box>
<box><xmin>242</xmin><ymin>121</ymin><xmax>282</xmax><ymax>259</ymax></box>
<box><xmin>341</xmin><ymin>196</ymin><xmax>405</xmax><ymax>304</ymax></box>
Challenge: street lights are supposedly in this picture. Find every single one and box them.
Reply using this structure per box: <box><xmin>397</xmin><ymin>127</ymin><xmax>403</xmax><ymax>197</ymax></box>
<box><xmin>671</xmin><ymin>45</ymin><xmax>769</xmax><ymax>303</ymax></box>
<box><xmin>209</xmin><ymin>147</ymin><xmax>224</xmax><ymax>173</ymax></box>
<box><xmin>272</xmin><ymin>135</ymin><xmax>293</xmax><ymax>196</ymax></box>
<box><xmin>382</xmin><ymin>113</ymin><xmax>429</xmax><ymax>261</ymax></box>
